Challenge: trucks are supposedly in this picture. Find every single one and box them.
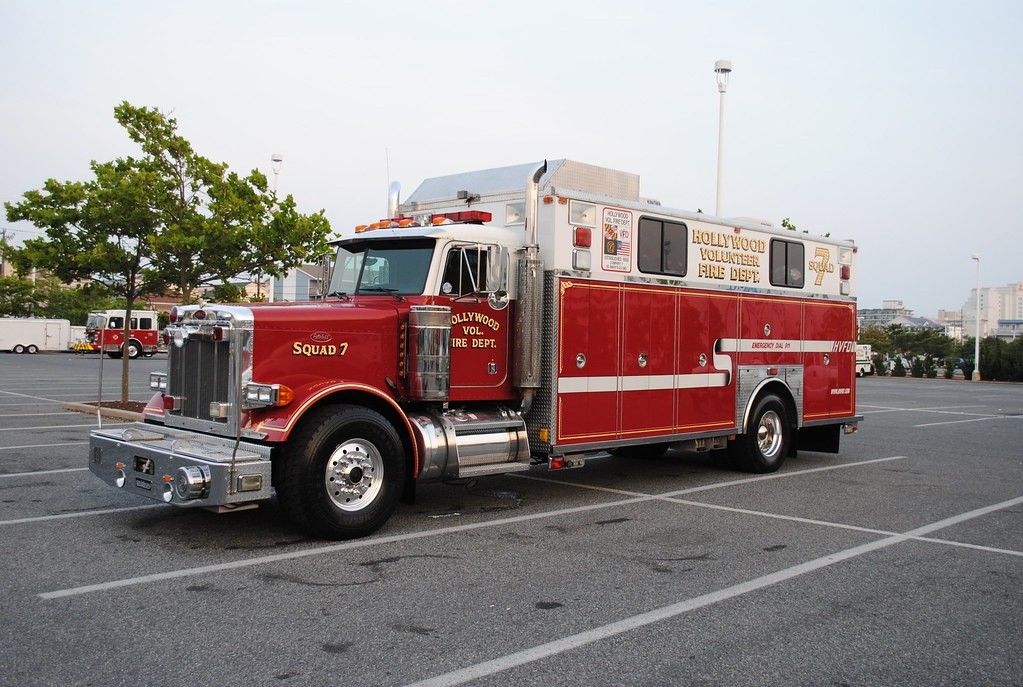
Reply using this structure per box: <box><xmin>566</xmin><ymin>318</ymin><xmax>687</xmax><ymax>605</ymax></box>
<box><xmin>0</xmin><ymin>320</ymin><xmax>70</xmax><ymax>354</ymax></box>
<box><xmin>71</xmin><ymin>326</ymin><xmax>88</xmax><ymax>349</ymax></box>
<box><xmin>855</xmin><ymin>346</ymin><xmax>871</xmax><ymax>377</ymax></box>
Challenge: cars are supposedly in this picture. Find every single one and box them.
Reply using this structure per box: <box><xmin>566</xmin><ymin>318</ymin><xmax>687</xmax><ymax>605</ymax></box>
<box><xmin>938</xmin><ymin>358</ymin><xmax>966</xmax><ymax>369</ymax></box>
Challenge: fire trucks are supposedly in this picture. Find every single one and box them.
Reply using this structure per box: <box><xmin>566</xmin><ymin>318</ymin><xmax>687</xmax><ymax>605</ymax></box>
<box><xmin>72</xmin><ymin>308</ymin><xmax>168</xmax><ymax>359</ymax></box>
<box><xmin>87</xmin><ymin>157</ymin><xmax>865</xmax><ymax>542</ymax></box>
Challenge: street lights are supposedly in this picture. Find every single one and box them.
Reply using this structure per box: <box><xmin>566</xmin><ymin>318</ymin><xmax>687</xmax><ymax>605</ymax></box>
<box><xmin>713</xmin><ymin>58</ymin><xmax>732</xmax><ymax>217</ymax></box>
<box><xmin>971</xmin><ymin>255</ymin><xmax>982</xmax><ymax>382</ymax></box>
<box><xmin>269</xmin><ymin>153</ymin><xmax>281</xmax><ymax>303</ymax></box>
